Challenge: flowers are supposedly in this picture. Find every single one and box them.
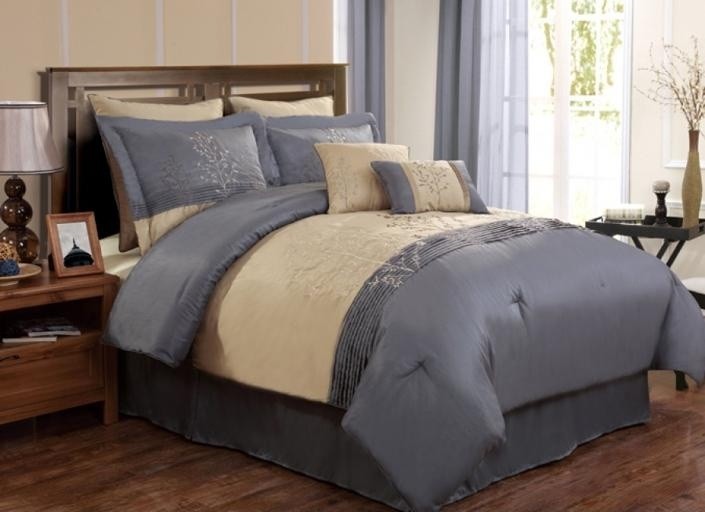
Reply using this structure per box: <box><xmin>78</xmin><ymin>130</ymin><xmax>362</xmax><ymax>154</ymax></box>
<box><xmin>634</xmin><ymin>35</ymin><xmax>704</xmax><ymax>128</ymax></box>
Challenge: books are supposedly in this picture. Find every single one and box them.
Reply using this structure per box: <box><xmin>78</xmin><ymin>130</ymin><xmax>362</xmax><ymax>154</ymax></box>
<box><xmin>20</xmin><ymin>316</ymin><xmax>82</xmax><ymax>337</ymax></box>
<box><xmin>2</xmin><ymin>329</ymin><xmax>57</xmax><ymax>343</ymax></box>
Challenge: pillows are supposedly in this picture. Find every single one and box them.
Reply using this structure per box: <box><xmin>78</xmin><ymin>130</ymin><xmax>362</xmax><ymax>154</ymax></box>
<box><xmin>86</xmin><ymin>90</ymin><xmax>226</xmax><ymax>254</ymax></box>
<box><xmin>312</xmin><ymin>141</ymin><xmax>414</xmax><ymax>216</ymax></box>
<box><xmin>370</xmin><ymin>158</ymin><xmax>494</xmax><ymax>217</ymax></box>
<box><xmin>263</xmin><ymin>111</ymin><xmax>381</xmax><ymax>187</ymax></box>
<box><xmin>227</xmin><ymin>94</ymin><xmax>336</xmax><ymax>118</ymax></box>
<box><xmin>94</xmin><ymin>108</ymin><xmax>280</xmax><ymax>255</ymax></box>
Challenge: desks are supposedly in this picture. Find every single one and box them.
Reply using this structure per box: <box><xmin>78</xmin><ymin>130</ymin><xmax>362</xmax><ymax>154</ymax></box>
<box><xmin>584</xmin><ymin>213</ymin><xmax>704</xmax><ymax>392</ymax></box>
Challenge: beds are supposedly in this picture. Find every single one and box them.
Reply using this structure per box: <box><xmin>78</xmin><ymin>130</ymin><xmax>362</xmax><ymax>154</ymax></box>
<box><xmin>44</xmin><ymin>61</ymin><xmax>704</xmax><ymax>510</ymax></box>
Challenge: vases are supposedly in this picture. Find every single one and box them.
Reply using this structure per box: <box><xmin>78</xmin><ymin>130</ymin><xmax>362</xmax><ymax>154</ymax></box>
<box><xmin>681</xmin><ymin>129</ymin><xmax>704</xmax><ymax>228</ymax></box>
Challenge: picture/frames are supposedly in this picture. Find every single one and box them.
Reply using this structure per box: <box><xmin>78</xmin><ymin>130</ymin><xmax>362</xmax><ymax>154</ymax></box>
<box><xmin>44</xmin><ymin>211</ymin><xmax>106</xmax><ymax>280</ymax></box>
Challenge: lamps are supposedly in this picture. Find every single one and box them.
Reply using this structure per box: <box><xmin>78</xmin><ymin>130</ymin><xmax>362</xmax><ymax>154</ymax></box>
<box><xmin>0</xmin><ymin>99</ymin><xmax>66</xmax><ymax>262</ymax></box>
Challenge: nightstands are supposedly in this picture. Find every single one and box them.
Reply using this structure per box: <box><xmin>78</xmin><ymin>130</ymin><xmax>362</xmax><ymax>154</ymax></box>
<box><xmin>1</xmin><ymin>265</ymin><xmax>121</xmax><ymax>432</ymax></box>
